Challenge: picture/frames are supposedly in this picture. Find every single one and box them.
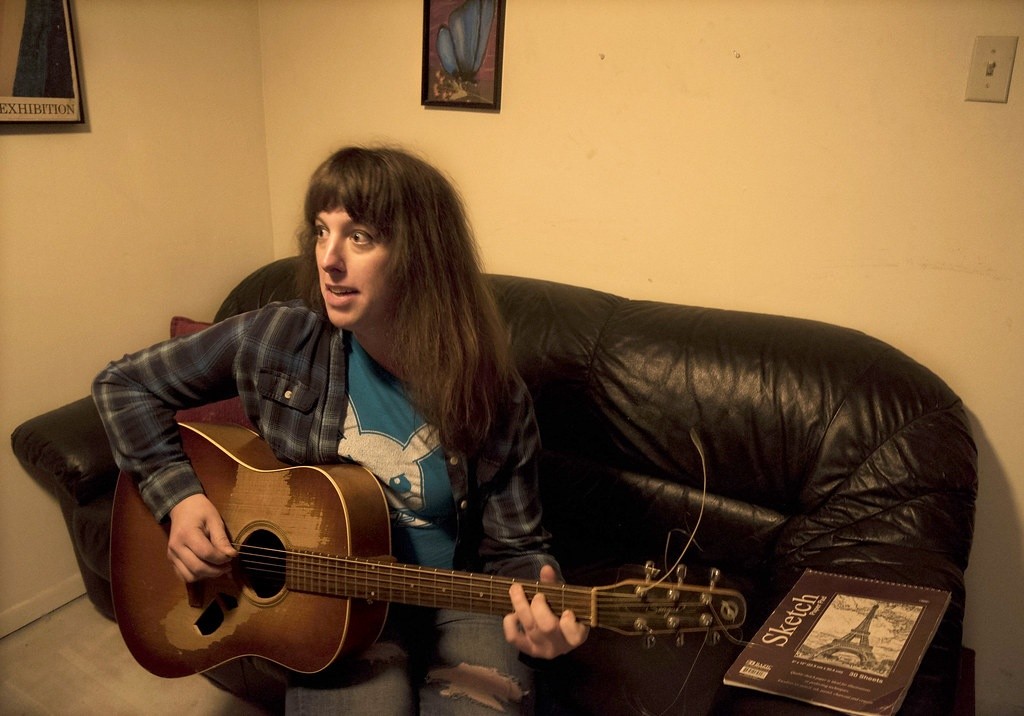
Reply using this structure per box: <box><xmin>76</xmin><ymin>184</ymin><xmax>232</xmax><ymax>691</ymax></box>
<box><xmin>0</xmin><ymin>0</ymin><xmax>87</xmax><ymax>127</ymax></box>
<box><xmin>420</xmin><ymin>0</ymin><xmax>508</xmax><ymax>113</ymax></box>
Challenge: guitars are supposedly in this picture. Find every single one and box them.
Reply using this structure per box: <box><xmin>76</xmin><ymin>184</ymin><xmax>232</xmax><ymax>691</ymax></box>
<box><xmin>110</xmin><ymin>420</ymin><xmax>748</xmax><ymax>678</ymax></box>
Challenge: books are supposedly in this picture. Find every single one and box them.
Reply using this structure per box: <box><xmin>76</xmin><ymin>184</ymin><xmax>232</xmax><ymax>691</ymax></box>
<box><xmin>721</xmin><ymin>568</ymin><xmax>954</xmax><ymax>716</ymax></box>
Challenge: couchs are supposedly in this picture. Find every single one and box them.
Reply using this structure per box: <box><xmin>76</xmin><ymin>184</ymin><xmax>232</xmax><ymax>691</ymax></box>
<box><xmin>7</xmin><ymin>254</ymin><xmax>978</xmax><ymax>715</ymax></box>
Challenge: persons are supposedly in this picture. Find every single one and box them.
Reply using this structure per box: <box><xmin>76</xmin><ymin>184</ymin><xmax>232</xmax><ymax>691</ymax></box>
<box><xmin>89</xmin><ymin>145</ymin><xmax>591</xmax><ymax>716</ymax></box>
